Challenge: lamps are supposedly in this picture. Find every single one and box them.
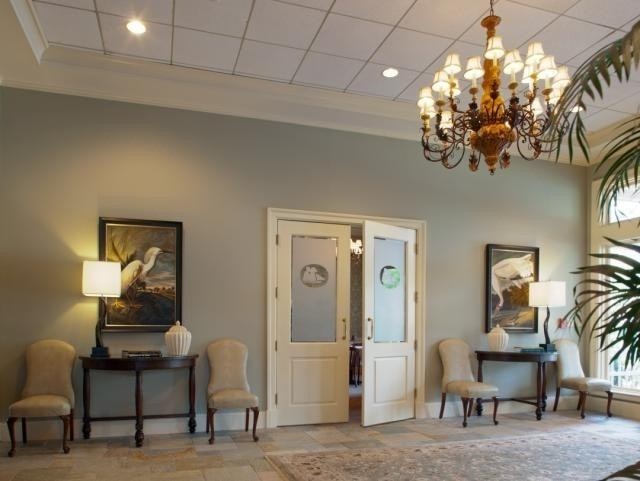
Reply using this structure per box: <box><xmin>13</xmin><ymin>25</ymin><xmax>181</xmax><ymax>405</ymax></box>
<box><xmin>349</xmin><ymin>236</ymin><xmax>362</xmax><ymax>260</ymax></box>
<box><xmin>82</xmin><ymin>261</ymin><xmax>120</xmax><ymax>356</ymax></box>
<box><xmin>415</xmin><ymin>0</ymin><xmax>584</xmax><ymax>174</ymax></box>
<box><xmin>527</xmin><ymin>279</ymin><xmax>566</xmax><ymax>351</ymax></box>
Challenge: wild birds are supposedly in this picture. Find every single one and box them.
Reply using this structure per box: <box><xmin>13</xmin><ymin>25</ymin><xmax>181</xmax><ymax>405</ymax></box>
<box><xmin>110</xmin><ymin>246</ymin><xmax>174</xmax><ymax>311</ymax></box>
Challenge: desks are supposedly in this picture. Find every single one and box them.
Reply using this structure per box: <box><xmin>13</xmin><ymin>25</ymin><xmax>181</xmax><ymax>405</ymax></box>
<box><xmin>350</xmin><ymin>343</ymin><xmax>363</xmax><ymax>388</ymax></box>
<box><xmin>475</xmin><ymin>349</ymin><xmax>557</xmax><ymax>419</ymax></box>
<box><xmin>79</xmin><ymin>348</ymin><xmax>198</xmax><ymax>443</ymax></box>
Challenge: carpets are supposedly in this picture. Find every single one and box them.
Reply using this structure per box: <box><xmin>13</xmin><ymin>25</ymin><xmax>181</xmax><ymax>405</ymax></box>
<box><xmin>262</xmin><ymin>427</ymin><xmax>640</xmax><ymax>481</ymax></box>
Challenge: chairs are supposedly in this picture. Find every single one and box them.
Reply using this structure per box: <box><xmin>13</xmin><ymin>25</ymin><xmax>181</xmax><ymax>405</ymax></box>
<box><xmin>553</xmin><ymin>338</ymin><xmax>613</xmax><ymax>418</ymax></box>
<box><xmin>439</xmin><ymin>337</ymin><xmax>498</xmax><ymax>426</ymax></box>
<box><xmin>206</xmin><ymin>339</ymin><xmax>261</xmax><ymax>443</ymax></box>
<box><xmin>8</xmin><ymin>339</ymin><xmax>76</xmax><ymax>455</ymax></box>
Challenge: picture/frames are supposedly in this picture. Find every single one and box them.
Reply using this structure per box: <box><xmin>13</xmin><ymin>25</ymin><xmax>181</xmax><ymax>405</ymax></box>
<box><xmin>98</xmin><ymin>217</ymin><xmax>184</xmax><ymax>332</ymax></box>
<box><xmin>485</xmin><ymin>242</ymin><xmax>540</xmax><ymax>333</ymax></box>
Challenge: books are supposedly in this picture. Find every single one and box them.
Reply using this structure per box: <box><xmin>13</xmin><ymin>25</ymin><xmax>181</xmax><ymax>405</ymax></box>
<box><xmin>121</xmin><ymin>349</ymin><xmax>162</xmax><ymax>357</ymax></box>
<box><xmin>513</xmin><ymin>346</ymin><xmax>544</xmax><ymax>352</ymax></box>
